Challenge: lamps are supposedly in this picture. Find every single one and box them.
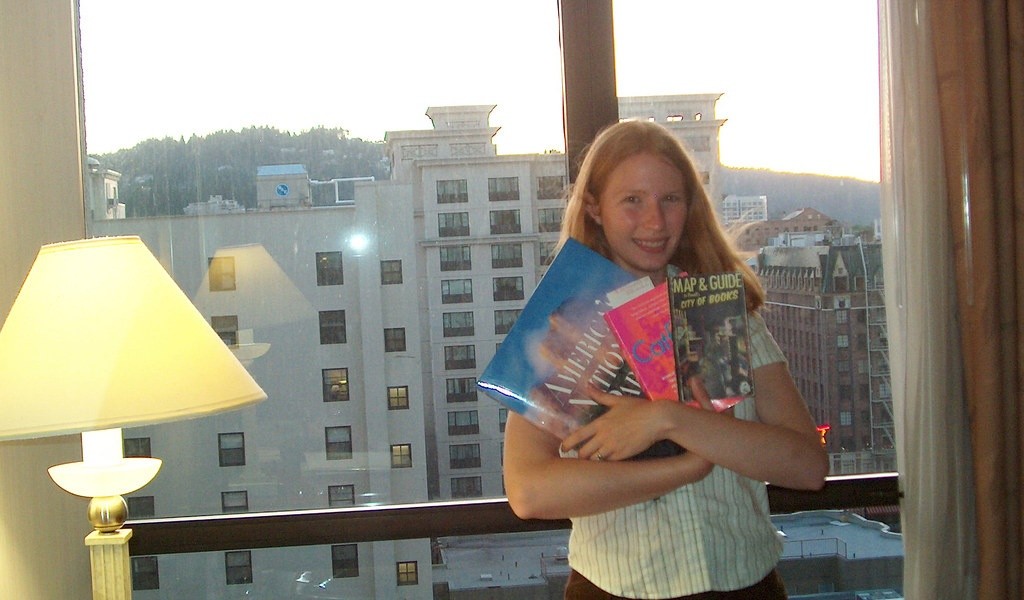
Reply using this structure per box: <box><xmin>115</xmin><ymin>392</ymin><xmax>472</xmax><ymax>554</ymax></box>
<box><xmin>0</xmin><ymin>234</ymin><xmax>268</xmax><ymax>599</ymax></box>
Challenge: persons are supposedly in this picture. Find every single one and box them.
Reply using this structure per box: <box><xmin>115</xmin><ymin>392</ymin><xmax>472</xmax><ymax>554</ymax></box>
<box><xmin>503</xmin><ymin>118</ymin><xmax>829</xmax><ymax>599</ymax></box>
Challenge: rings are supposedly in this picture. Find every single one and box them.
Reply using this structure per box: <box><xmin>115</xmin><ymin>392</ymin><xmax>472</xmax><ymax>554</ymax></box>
<box><xmin>595</xmin><ymin>451</ymin><xmax>605</xmax><ymax>461</ymax></box>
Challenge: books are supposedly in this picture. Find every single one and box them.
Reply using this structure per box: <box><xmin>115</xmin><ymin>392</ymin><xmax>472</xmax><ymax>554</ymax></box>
<box><xmin>477</xmin><ymin>236</ymin><xmax>754</xmax><ymax>462</ymax></box>
